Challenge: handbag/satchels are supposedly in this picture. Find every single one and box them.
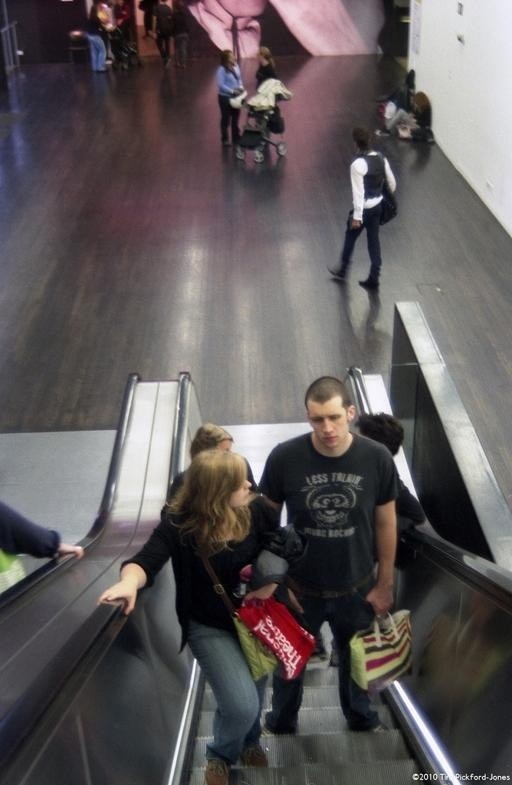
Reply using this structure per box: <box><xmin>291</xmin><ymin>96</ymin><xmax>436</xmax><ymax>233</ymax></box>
<box><xmin>350</xmin><ymin>609</ymin><xmax>415</xmax><ymax>693</ymax></box>
<box><xmin>231</xmin><ymin>596</ymin><xmax>316</xmax><ymax>683</ymax></box>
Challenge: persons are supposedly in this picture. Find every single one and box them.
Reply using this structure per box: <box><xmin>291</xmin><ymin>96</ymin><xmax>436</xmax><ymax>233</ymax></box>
<box><xmin>85</xmin><ymin>0</ymin><xmax>190</xmax><ymax>72</ymax></box>
<box><xmin>172</xmin><ymin>0</ymin><xmax>374</xmax><ymax>55</ymax></box>
<box><xmin>326</xmin><ymin>125</ymin><xmax>398</xmax><ymax>290</ymax></box>
<box><xmin>256</xmin><ymin>46</ymin><xmax>276</xmax><ymax>91</ymax></box>
<box><xmin>95</xmin><ymin>377</ymin><xmax>426</xmax><ymax>785</ymax></box>
<box><xmin>0</xmin><ymin>497</ymin><xmax>85</xmax><ymax>593</ymax></box>
<box><xmin>216</xmin><ymin>50</ymin><xmax>245</xmax><ymax>153</ymax></box>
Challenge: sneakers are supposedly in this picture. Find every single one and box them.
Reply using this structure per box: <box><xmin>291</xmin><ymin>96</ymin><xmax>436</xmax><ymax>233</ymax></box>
<box><xmin>205</xmin><ymin>753</ymin><xmax>232</xmax><ymax>785</ymax></box>
<box><xmin>241</xmin><ymin>744</ymin><xmax>269</xmax><ymax>768</ymax></box>
<box><xmin>358</xmin><ymin>276</ymin><xmax>379</xmax><ymax>289</ymax></box>
<box><xmin>325</xmin><ymin>265</ymin><xmax>347</xmax><ymax>279</ymax></box>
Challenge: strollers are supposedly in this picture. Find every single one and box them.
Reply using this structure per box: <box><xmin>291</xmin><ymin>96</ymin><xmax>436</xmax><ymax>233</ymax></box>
<box><xmin>107</xmin><ymin>24</ymin><xmax>145</xmax><ymax>72</ymax></box>
<box><xmin>233</xmin><ymin>100</ymin><xmax>289</xmax><ymax>166</ymax></box>
